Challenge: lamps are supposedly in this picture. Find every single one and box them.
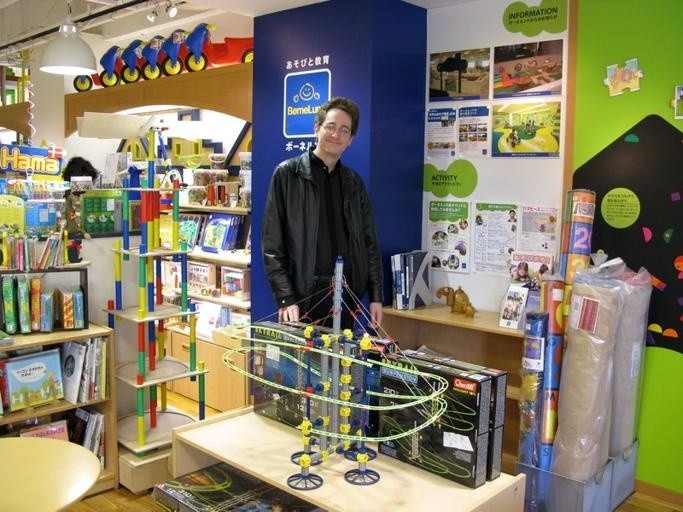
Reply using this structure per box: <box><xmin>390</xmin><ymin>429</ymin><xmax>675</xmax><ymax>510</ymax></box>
<box><xmin>147</xmin><ymin>1</ymin><xmax>178</xmax><ymax>24</ymax></box>
<box><xmin>39</xmin><ymin>0</ymin><xmax>98</xmax><ymax>76</ymax></box>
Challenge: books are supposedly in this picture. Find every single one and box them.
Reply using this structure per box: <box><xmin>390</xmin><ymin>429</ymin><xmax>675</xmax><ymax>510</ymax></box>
<box><xmin>390</xmin><ymin>250</ymin><xmax>434</xmax><ymax>311</ymax></box>
<box><xmin>64</xmin><ymin>336</ymin><xmax>109</xmax><ymax>405</ymax></box>
<box><xmin>178</xmin><ymin>213</ymin><xmax>245</xmax><ymax>253</ymax></box>
<box><xmin>63</xmin><ymin>406</ymin><xmax>106</xmax><ymax>470</ymax></box>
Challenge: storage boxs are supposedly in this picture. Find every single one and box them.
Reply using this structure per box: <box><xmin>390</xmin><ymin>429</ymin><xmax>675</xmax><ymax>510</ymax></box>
<box><xmin>515</xmin><ymin>438</ymin><xmax>638</xmax><ymax>512</ymax></box>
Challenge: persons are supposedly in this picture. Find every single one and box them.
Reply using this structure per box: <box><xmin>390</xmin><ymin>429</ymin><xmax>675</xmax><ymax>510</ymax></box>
<box><xmin>62</xmin><ymin>156</ymin><xmax>99</xmax><ymax>187</ymax></box>
<box><xmin>261</xmin><ymin>97</ymin><xmax>384</xmax><ymax>329</ymax></box>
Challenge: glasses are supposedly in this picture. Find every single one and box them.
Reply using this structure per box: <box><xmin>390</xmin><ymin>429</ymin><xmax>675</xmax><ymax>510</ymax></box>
<box><xmin>320</xmin><ymin>124</ymin><xmax>351</xmax><ymax>135</ymax></box>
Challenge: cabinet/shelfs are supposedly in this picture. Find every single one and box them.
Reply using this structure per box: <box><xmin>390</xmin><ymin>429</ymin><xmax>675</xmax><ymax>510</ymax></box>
<box><xmin>173</xmin><ymin>405</ymin><xmax>525</xmax><ymax>511</ymax></box>
<box><xmin>0</xmin><ymin>145</ymin><xmax>252</xmax><ymax>499</ymax></box>
<box><xmin>381</xmin><ymin>302</ymin><xmax>524</xmax><ymax>477</ymax></box>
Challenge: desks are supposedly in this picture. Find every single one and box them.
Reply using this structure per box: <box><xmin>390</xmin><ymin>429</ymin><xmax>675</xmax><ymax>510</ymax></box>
<box><xmin>0</xmin><ymin>437</ymin><xmax>101</xmax><ymax>512</ymax></box>
